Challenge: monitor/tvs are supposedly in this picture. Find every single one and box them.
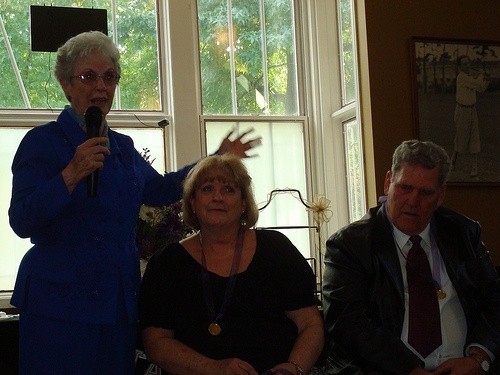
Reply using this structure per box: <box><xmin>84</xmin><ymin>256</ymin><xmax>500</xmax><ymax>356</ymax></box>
<box><xmin>30</xmin><ymin>4</ymin><xmax>109</xmax><ymax>53</ymax></box>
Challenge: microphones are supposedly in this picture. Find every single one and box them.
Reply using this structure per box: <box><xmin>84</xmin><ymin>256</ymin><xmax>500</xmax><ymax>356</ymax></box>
<box><xmin>85</xmin><ymin>105</ymin><xmax>104</xmax><ymax>196</ymax></box>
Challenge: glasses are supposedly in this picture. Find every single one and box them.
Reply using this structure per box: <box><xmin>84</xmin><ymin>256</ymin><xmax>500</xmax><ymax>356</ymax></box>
<box><xmin>70</xmin><ymin>69</ymin><xmax>120</xmax><ymax>87</ymax></box>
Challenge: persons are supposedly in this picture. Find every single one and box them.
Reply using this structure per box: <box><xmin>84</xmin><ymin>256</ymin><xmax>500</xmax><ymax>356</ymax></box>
<box><xmin>138</xmin><ymin>154</ymin><xmax>326</xmax><ymax>375</ymax></box>
<box><xmin>322</xmin><ymin>140</ymin><xmax>500</xmax><ymax>375</ymax></box>
<box><xmin>8</xmin><ymin>32</ymin><xmax>263</xmax><ymax>375</ymax></box>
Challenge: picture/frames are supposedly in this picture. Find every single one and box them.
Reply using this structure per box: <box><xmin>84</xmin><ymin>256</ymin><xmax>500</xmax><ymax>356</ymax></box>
<box><xmin>408</xmin><ymin>36</ymin><xmax>500</xmax><ymax>189</ymax></box>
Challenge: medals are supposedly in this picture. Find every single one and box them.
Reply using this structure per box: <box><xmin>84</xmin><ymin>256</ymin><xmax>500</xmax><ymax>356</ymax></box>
<box><xmin>208</xmin><ymin>321</ymin><xmax>221</xmax><ymax>336</ymax></box>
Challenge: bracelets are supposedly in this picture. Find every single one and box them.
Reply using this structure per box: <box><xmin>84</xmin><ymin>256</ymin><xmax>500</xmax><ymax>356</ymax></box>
<box><xmin>287</xmin><ymin>360</ymin><xmax>303</xmax><ymax>375</ymax></box>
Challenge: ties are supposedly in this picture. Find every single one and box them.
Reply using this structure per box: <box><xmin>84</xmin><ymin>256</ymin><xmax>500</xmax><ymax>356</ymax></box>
<box><xmin>406</xmin><ymin>235</ymin><xmax>443</xmax><ymax>360</ymax></box>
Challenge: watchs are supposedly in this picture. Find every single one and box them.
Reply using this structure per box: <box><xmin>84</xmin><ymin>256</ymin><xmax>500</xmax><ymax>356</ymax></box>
<box><xmin>468</xmin><ymin>353</ymin><xmax>491</xmax><ymax>375</ymax></box>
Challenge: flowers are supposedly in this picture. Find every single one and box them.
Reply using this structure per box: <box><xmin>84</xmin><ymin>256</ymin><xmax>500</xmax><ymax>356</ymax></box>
<box><xmin>138</xmin><ymin>202</ymin><xmax>193</xmax><ymax>255</ymax></box>
